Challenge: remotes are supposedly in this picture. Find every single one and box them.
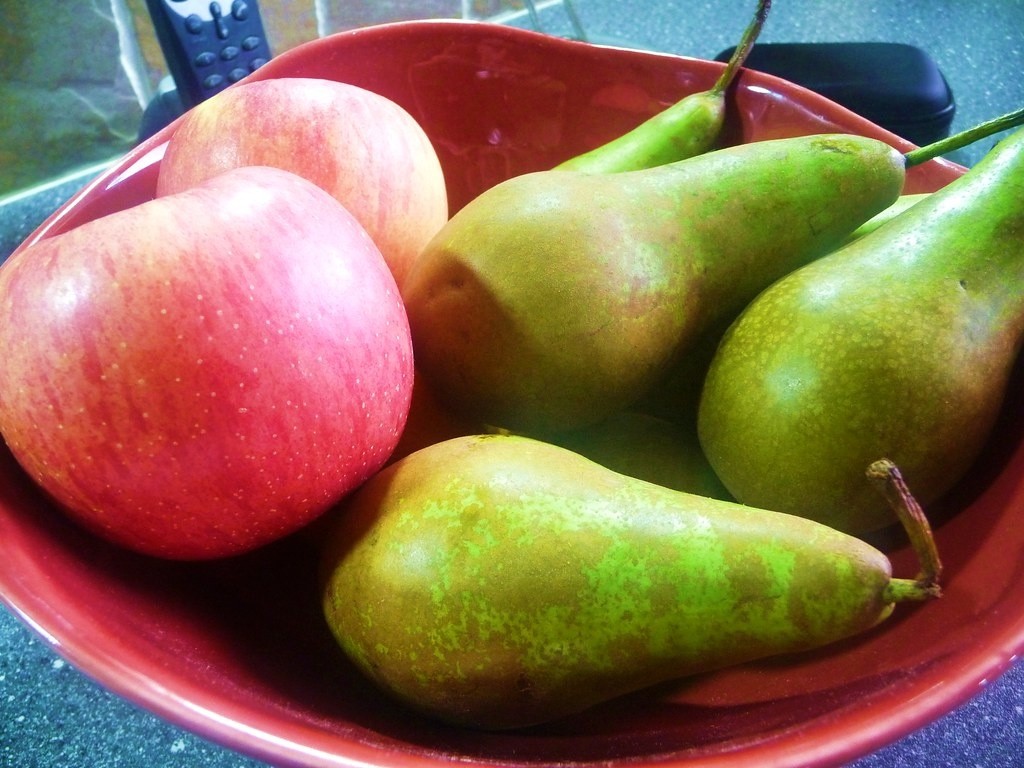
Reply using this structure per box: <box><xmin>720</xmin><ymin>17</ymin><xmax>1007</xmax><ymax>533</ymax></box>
<box><xmin>145</xmin><ymin>0</ymin><xmax>274</xmax><ymax>111</ymax></box>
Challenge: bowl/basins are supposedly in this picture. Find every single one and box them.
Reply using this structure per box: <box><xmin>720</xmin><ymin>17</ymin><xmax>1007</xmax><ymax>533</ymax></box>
<box><xmin>0</xmin><ymin>20</ymin><xmax>1024</xmax><ymax>768</ymax></box>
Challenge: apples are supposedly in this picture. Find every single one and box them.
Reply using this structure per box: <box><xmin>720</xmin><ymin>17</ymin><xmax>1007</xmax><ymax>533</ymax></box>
<box><xmin>155</xmin><ymin>77</ymin><xmax>448</xmax><ymax>292</ymax></box>
<box><xmin>0</xmin><ymin>166</ymin><xmax>414</xmax><ymax>557</ymax></box>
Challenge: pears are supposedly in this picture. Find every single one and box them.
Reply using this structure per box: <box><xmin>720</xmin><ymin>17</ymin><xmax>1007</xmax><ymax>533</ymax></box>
<box><xmin>315</xmin><ymin>0</ymin><xmax>1024</xmax><ymax>730</ymax></box>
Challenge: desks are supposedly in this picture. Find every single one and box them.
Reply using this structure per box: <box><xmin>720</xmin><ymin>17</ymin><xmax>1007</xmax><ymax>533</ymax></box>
<box><xmin>0</xmin><ymin>0</ymin><xmax>1024</xmax><ymax>768</ymax></box>
